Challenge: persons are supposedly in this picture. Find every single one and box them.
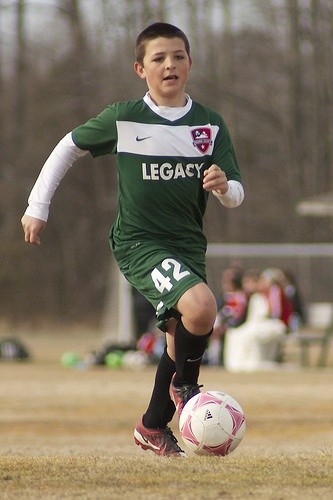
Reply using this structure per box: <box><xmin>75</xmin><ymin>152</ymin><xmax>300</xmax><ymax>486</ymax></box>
<box><xmin>131</xmin><ymin>258</ymin><xmax>310</xmax><ymax>371</ymax></box>
<box><xmin>20</xmin><ymin>23</ymin><xmax>246</xmax><ymax>460</ymax></box>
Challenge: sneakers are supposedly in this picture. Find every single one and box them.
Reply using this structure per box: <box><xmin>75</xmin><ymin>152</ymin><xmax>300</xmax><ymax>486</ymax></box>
<box><xmin>170</xmin><ymin>370</ymin><xmax>202</xmax><ymax>416</ymax></box>
<box><xmin>133</xmin><ymin>413</ymin><xmax>187</xmax><ymax>458</ymax></box>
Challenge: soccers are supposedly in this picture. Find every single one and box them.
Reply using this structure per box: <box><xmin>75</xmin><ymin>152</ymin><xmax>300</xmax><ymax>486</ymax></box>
<box><xmin>178</xmin><ymin>390</ymin><xmax>247</xmax><ymax>457</ymax></box>
